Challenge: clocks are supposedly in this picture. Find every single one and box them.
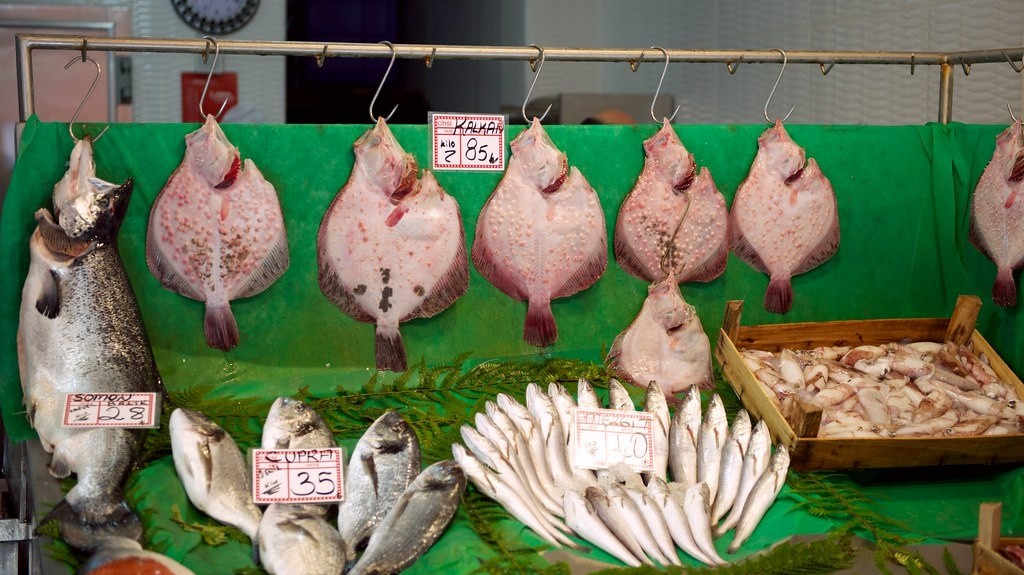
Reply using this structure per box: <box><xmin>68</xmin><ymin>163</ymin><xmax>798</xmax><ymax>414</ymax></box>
<box><xmin>171</xmin><ymin>0</ymin><xmax>260</xmax><ymax>36</ymax></box>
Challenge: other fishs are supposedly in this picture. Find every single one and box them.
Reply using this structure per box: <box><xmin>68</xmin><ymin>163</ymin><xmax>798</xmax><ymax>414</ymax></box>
<box><xmin>14</xmin><ymin>110</ymin><xmax>1024</xmax><ymax>574</ymax></box>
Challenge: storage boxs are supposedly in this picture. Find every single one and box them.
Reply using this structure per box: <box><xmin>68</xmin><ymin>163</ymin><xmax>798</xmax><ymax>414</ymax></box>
<box><xmin>973</xmin><ymin>501</ymin><xmax>1023</xmax><ymax>575</ymax></box>
<box><xmin>711</xmin><ymin>293</ymin><xmax>1024</xmax><ymax>476</ymax></box>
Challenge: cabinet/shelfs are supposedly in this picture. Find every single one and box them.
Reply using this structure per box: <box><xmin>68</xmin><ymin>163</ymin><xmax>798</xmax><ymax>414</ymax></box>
<box><xmin>501</xmin><ymin>90</ymin><xmax>676</xmax><ymax>125</ymax></box>
